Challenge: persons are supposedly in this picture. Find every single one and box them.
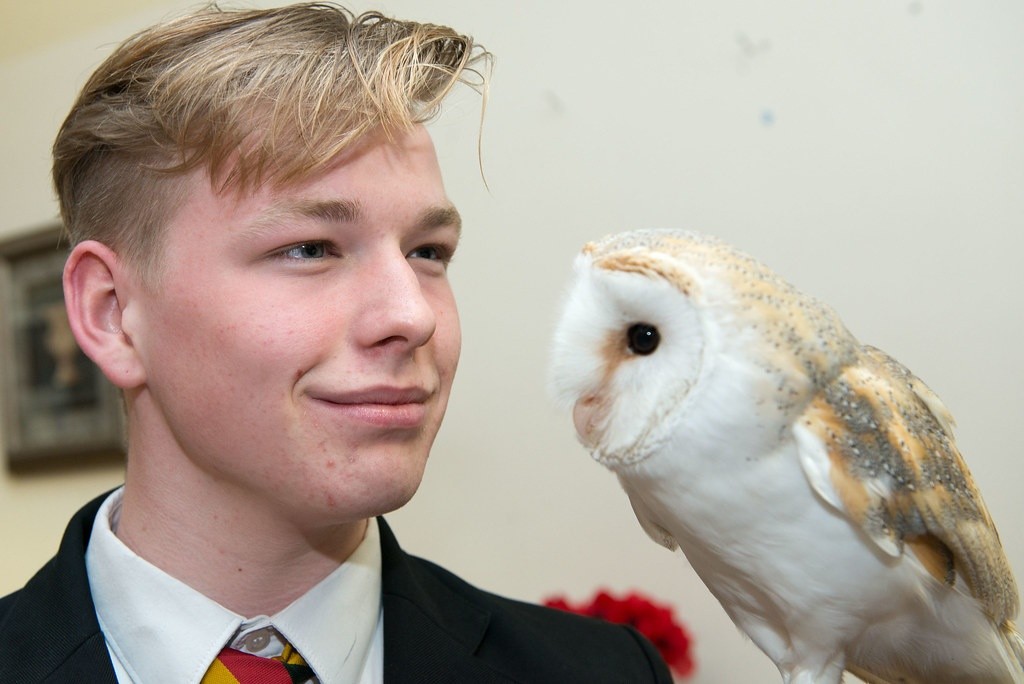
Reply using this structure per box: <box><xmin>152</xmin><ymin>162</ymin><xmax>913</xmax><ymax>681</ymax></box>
<box><xmin>0</xmin><ymin>2</ymin><xmax>673</xmax><ymax>684</ymax></box>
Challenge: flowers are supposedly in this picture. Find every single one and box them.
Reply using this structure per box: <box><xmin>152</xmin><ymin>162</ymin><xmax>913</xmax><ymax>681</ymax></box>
<box><xmin>541</xmin><ymin>589</ymin><xmax>692</xmax><ymax>679</ymax></box>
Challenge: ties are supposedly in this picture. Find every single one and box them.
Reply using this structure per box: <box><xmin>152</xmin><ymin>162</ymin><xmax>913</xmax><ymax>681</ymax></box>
<box><xmin>200</xmin><ymin>642</ymin><xmax>315</xmax><ymax>684</ymax></box>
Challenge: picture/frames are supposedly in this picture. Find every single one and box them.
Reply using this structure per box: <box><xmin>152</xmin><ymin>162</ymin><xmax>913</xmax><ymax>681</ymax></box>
<box><xmin>0</xmin><ymin>228</ymin><xmax>126</xmax><ymax>470</ymax></box>
